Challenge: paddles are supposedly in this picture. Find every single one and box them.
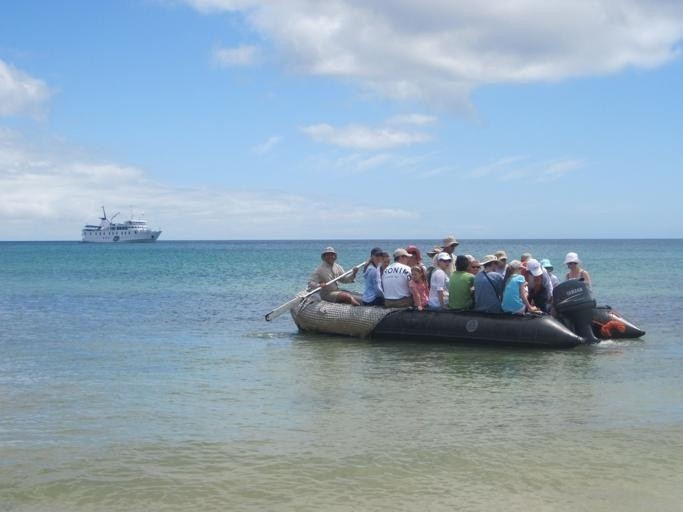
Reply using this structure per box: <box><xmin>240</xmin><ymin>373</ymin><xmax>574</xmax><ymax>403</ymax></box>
<box><xmin>265</xmin><ymin>261</ymin><xmax>367</xmax><ymax>321</ymax></box>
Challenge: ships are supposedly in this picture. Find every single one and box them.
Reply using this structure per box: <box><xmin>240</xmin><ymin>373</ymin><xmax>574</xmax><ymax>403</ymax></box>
<box><xmin>81</xmin><ymin>204</ymin><xmax>164</xmax><ymax>243</ymax></box>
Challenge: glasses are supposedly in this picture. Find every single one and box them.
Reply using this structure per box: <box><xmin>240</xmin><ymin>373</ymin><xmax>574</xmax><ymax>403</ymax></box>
<box><xmin>472</xmin><ymin>266</ymin><xmax>480</xmax><ymax>268</ymax></box>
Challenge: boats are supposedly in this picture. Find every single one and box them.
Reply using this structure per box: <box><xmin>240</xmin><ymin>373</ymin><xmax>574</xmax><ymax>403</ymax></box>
<box><xmin>290</xmin><ymin>288</ymin><xmax>647</xmax><ymax>349</ymax></box>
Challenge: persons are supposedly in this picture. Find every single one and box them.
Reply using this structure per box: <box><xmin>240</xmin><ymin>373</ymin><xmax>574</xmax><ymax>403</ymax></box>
<box><xmin>501</xmin><ymin>260</ymin><xmax>537</xmax><ymax>314</ymax></box>
<box><xmin>521</xmin><ymin>253</ymin><xmax>534</xmax><ymax>313</ymax></box>
<box><xmin>448</xmin><ymin>255</ymin><xmax>475</xmax><ymax>311</ymax></box>
<box><xmin>469</xmin><ymin>260</ymin><xmax>481</xmax><ymax>300</ymax></box>
<box><xmin>464</xmin><ymin>254</ymin><xmax>474</xmax><ymax>272</ymax></box>
<box><xmin>308</xmin><ymin>247</ymin><xmax>360</xmax><ymax>305</ymax></box>
<box><xmin>494</xmin><ymin>251</ymin><xmax>509</xmax><ymax>279</ymax></box>
<box><xmin>440</xmin><ymin>236</ymin><xmax>460</xmax><ymax>277</ymax></box>
<box><xmin>524</xmin><ymin>259</ymin><xmax>554</xmax><ymax>313</ymax></box>
<box><xmin>474</xmin><ymin>255</ymin><xmax>504</xmax><ymax>314</ymax></box>
<box><xmin>379</xmin><ymin>252</ymin><xmax>390</xmax><ymax>292</ymax></box>
<box><xmin>428</xmin><ymin>252</ymin><xmax>451</xmax><ymax>310</ymax></box>
<box><xmin>427</xmin><ymin>246</ymin><xmax>444</xmax><ymax>290</ymax></box>
<box><xmin>363</xmin><ymin>248</ymin><xmax>385</xmax><ymax>306</ymax></box>
<box><xmin>382</xmin><ymin>248</ymin><xmax>413</xmax><ymax>308</ymax></box>
<box><xmin>563</xmin><ymin>252</ymin><xmax>592</xmax><ymax>334</ymax></box>
<box><xmin>409</xmin><ymin>266</ymin><xmax>430</xmax><ymax>311</ymax></box>
<box><xmin>541</xmin><ymin>259</ymin><xmax>559</xmax><ymax>312</ymax></box>
<box><xmin>406</xmin><ymin>246</ymin><xmax>429</xmax><ymax>287</ymax></box>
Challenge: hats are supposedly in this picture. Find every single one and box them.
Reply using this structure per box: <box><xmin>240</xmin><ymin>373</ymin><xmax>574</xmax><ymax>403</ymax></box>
<box><xmin>563</xmin><ymin>253</ymin><xmax>580</xmax><ymax>266</ymax></box>
<box><xmin>371</xmin><ymin>247</ymin><xmax>383</xmax><ymax>255</ymax></box>
<box><xmin>540</xmin><ymin>259</ymin><xmax>554</xmax><ymax>272</ymax></box>
<box><xmin>405</xmin><ymin>246</ymin><xmax>422</xmax><ymax>261</ymax></box>
<box><xmin>494</xmin><ymin>251</ymin><xmax>507</xmax><ymax>262</ymax></box>
<box><xmin>427</xmin><ymin>248</ymin><xmax>441</xmax><ymax>257</ymax></box>
<box><xmin>478</xmin><ymin>255</ymin><xmax>498</xmax><ymax>266</ymax></box>
<box><xmin>510</xmin><ymin>261</ymin><xmax>523</xmax><ymax>269</ymax></box>
<box><xmin>320</xmin><ymin>246</ymin><xmax>336</xmax><ymax>259</ymax></box>
<box><xmin>438</xmin><ymin>252</ymin><xmax>452</xmax><ymax>262</ymax></box>
<box><xmin>526</xmin><ymin>259</ymin><xmax>543</xmax><ymax>278</ymax></box>
<box><xmin>442</xmin><ymin>235</ymin><xmax>459</xmax><ymax>251</ymax></box>
<box><xmin>393</xmin><ymin>248</ymin><xmax>412</xmax><ymax>259</ymax></box>
